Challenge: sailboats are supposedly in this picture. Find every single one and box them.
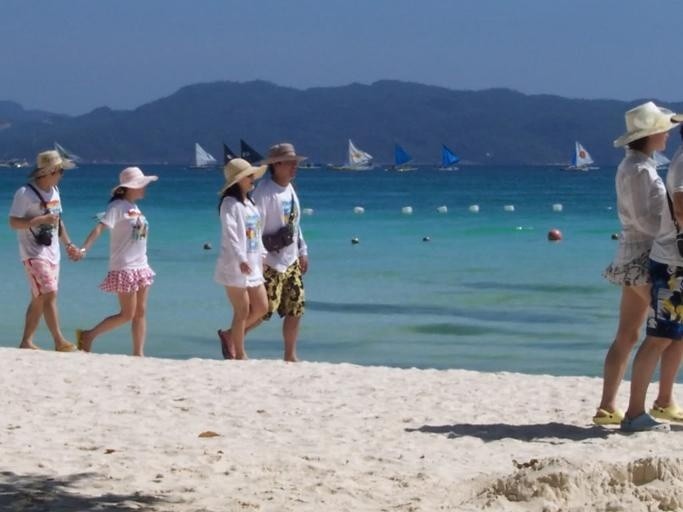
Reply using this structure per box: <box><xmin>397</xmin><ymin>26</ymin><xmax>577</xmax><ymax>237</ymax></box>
<box><xmin>51</xmin><ymin>142</ymin><xmax>79</xmax><ymax>170</ymax></box>
<box><xmin>652</xmin><ymin>150</ymin><xmax>672</xmax><ymax>170</ymax></box>
<box><xmin>187</xmin><ymin>137</ymin><xmax>461</xmax><ymax>173</ymax></box>
<box><xmin>558</xmin><ymin>141</ymin><xmax>600</xmax><ymax>173</ymax></box>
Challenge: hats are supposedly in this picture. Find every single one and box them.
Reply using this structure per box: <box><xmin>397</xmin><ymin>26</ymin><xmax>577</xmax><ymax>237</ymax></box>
<box><xmin>110</xmin><ymin>167</ymin><xmax>159</xmax><ymax>198</ymax></box>
<box><xmin>256</xmin><ymin>143</ymin><xmax>308</xmax><ymax>165</ymax></box>
<box><xmin>613</xmin><ymin>101</ymin><xmax>680</xmax><ymax>149</ymax></box>
<box><xmin>27</xmin><ymin>151</ymin><xmax>73</xmax><ymax>179</ymax></box>
<box><xmin>222</xmin><ymin>158</ymin><xmax>268</xmax><ymax>195</ymax></box>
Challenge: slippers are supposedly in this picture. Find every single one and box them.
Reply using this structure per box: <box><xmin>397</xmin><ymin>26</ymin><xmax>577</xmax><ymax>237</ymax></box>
<box><xmin>77</xmin><ymin>329</ymin><xmax>90</xmax><ymax>352</ymax></box>
<box><xmin>56</xmin><ymin>344</ymin><xmax>80</xmax><ymax>352</ymax></box>
<box><xmin>218</xmin><ymin>328</ymin><xmax>236</xmax><ymax>359</ymax></box>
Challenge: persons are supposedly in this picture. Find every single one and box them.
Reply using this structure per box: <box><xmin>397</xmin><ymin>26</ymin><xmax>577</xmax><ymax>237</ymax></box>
<box><xmin>243</xmin><ymin>141</ymin><xmax>310</xmax><ymax>363</ymax></box>
<box><xmin>590</xmin><ymin>97</ymin><xmax>682</xmax><ymax>426</ymax></box>
<box><xmin>619</xmin><ymin>113</ymin><xmax>682</xmax><ymax>434</ymax></box>
<box><xmin>7</xmin><ymin>150</ymin><xmax>78</xmax><ymax>352</ymax></box>
<box><xmin>211</xmin><ymin>155</ymin><xmax>270</xmax><ymax>361</ymax></box>
<box><xmin>68</xmin><ymin>166</ymin><xmax>158</xmax><ymax>359</ymax></box>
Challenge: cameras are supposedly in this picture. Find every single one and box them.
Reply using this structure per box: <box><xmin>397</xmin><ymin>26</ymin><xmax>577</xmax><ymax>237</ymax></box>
<box><xmin>35</xmin><ymin>228</ymin><xmax>52</xmax><ymax>245</ymax></box>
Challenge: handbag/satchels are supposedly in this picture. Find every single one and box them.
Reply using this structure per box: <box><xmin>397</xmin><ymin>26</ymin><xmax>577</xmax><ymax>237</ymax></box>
<box><xmin>261</xmin><ymin>222</ymin><xmax>293</xmax><ymax>254</ymax></box>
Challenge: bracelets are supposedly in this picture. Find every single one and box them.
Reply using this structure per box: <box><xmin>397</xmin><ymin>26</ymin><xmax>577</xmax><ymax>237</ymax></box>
<box><xmin>79</xmin><ymin>247</ymin><xmax>85</xmax><ymax>256</ymax></box>
<box><xmin>64</xmin><ymin>240</ymin><xmax>72</xmax><ymax>249</ymax></box>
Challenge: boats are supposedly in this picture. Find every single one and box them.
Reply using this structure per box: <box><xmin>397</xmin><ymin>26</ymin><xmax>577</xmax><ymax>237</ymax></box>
<box><xmin>2</xmin><ymin>158</ymin><xmax>30</xmax><ymax>168</ymax></box>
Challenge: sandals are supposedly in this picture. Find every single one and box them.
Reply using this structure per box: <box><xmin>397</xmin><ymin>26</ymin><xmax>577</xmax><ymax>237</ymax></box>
<box><xmin>593</xmin><ymin>407</ymin><xmax>624</xmax><ymax>424</ymax></box>
<box><xmin>649</xmin><ymin>399</ymin><xmax>683</xmax><ymax>423</ymax></box>
<box><xmin>621</xmin><ymin>413</ymin><xmax>671</xmax><ymax>433</ymax></box>
